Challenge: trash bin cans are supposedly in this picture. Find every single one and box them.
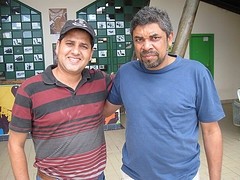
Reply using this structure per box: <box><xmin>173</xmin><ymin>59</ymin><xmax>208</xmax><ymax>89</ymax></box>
<box><xmin>233</xmin><ymin>99</ymin><xmax>240</xmax><ymax>126</ymax></box>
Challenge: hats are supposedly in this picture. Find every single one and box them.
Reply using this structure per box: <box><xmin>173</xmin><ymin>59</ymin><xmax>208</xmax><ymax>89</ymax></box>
<box><xmin>60</xmin><ymin>18</ymin><xmax>95</xmax><ymax>40</ymax></box>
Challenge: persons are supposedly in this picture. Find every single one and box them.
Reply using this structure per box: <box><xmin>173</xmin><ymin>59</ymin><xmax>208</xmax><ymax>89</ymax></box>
<box><xmin>101</xmin><ymin>4</ymin><xmax>226</xmax><ymax>179</ymax></box>
<box><xmin>7</xmin><ymin>18</ymin><xmax>118</xmax><ymax>180</ymax></box>
